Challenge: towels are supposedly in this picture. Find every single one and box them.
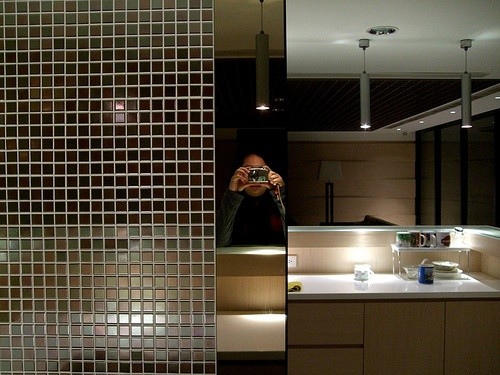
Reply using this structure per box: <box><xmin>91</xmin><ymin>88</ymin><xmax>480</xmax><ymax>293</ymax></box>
<box><xmin>353</xmin><ymin>281</ymin><xmax>370</xmax><ymax>291</ymax></box>
<box><xmin>288</xmin><ymin>281</ymin><xmax>303</xmax><ymax>292</ymax></box>
<box><xmin>354</xmin><ymin>264</ymin><xmax>374</xmax><ymax>280</ymax></box>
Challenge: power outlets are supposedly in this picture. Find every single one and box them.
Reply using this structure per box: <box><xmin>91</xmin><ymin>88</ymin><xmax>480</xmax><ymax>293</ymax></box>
<box><xmin>288</xmin><ymin>256</ymin><xmax>296</xmax><ymax>267</ymax></box>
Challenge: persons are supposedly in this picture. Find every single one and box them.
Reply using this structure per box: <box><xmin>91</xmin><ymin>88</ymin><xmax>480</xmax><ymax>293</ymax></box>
<box><xmin>215</xmin><ymin>149</ymin><xmax>286</xmax><ymax>248</ymax></box>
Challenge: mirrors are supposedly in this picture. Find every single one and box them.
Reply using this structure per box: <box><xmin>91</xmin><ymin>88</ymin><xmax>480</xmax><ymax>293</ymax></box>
<box><xmin>214</xmin><ymin>0</ymin><xmax>284</xmax><ymax>247</ymax></box>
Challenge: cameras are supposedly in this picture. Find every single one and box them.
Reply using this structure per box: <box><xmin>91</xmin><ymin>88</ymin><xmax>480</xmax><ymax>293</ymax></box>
<box><xmin>244</xmin><ymin>168</ymin><xmax>271</xmax><ymax>183</ymax></box>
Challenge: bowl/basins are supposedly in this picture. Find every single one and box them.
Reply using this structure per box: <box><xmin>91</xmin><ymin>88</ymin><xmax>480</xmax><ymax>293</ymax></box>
<box><xmin>402</xmin><ymin>266</ymin><xmax>418</xmax><ymax>279</ymax></box>
<box><xmin>433</xmin><ymin>260</ymin><xmax>459</xmax><ymax>271</ymax></box>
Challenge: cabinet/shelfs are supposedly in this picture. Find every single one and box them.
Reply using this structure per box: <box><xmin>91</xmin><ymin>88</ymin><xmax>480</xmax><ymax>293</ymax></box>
<box><xmin>287</xmin><ymin>300</ymin><xmax>500</xmax><ymax>375</ymax></box>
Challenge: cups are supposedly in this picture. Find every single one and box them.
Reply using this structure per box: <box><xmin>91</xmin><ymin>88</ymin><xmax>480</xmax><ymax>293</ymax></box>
<box><xmin>396</xmin><ymin>229</ymin><xmax>451</xmax><ymax>248</ymax></box>
<box><xmin>417</xmin><ymin>264</ymin><xmax>434</xmax><ymax>284</ymax></box>
<box><xmin>353</xmin><ymin>264</ymin><xmax>370</xmax><ymax>282</ymax></box>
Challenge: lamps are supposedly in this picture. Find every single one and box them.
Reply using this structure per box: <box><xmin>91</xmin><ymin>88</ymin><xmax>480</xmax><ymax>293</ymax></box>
<box><xmin>461</xmin><ymin>39</ymin><xmax>472</xmax><ymax>128</ymax></box>
<box><xmin>255</xmin><ymin>0</ymin><xmax>269</xmax><ymax>110</ymax></box>
<box><xmin>359</xmin><ymin>39</ymin><xmax>372</xmax><ymax>130</ymax></box>
